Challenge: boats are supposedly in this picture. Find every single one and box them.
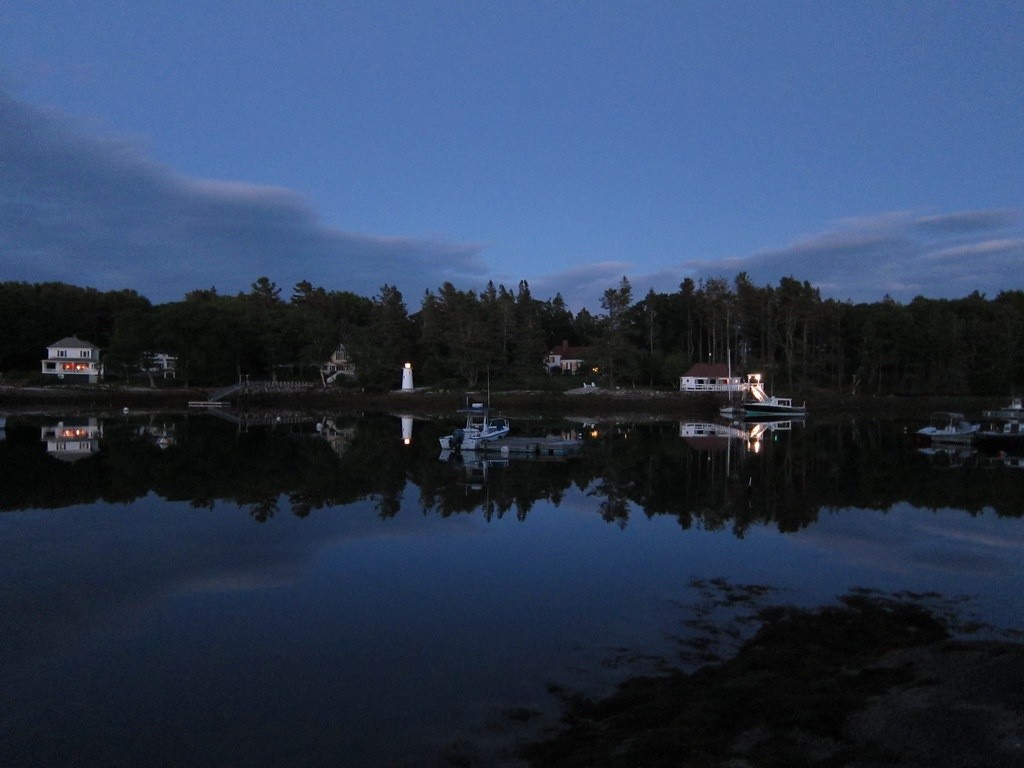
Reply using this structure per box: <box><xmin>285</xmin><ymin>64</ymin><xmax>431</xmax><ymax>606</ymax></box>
<box><xmin>437</xmin><ymin>360</ymin><xmax>511</xmax><ymax>451</ymax></box>
<box><xmin>914</xmin><ymin>411</ymin><xmax>981</xmax><ymax>439</ymax></box>
<box><xmin>974</xmin><ymin>397</ymin><xmax>1024</xmax><ymax>438</ymax></box>
<box><xmin>737</xmin><ymin>398</ymin><xmax>808</xmax><ymax>419</ymax></box>
